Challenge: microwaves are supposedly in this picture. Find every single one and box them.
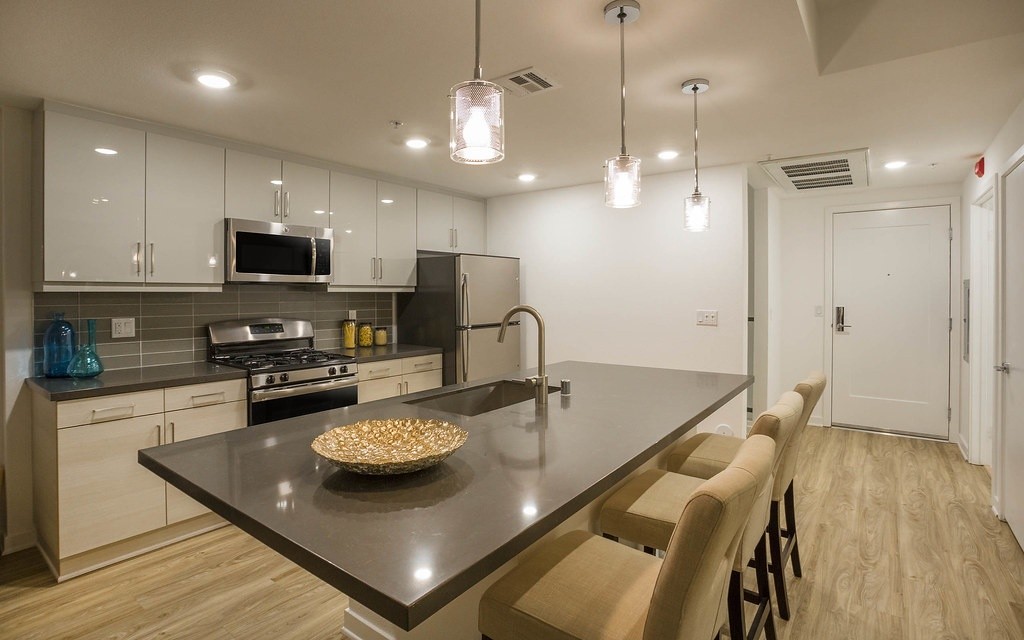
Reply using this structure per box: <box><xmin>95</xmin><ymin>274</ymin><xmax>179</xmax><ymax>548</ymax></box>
<box><xmin>225</xmin><ymin>218</ymin><xmax>334</xmax><ymax>285</ymax></box>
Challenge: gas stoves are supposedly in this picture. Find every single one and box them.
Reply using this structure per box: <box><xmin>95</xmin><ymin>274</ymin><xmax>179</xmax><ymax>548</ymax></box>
<box><xmin>206</xmin><ymin>317</ymin><xmax>358</xmax><ymax>388</ymax></box>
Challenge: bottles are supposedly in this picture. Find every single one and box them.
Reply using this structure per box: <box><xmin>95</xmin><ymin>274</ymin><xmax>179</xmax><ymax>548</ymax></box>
<box><xmin>42</xmin><ymin>312</ymin><xmax>76</xmax><ymax>377</ymax></box>
<box><xmin>374</xmin><ymin>327</ymin><xmax>387</xmax><ymax>346</ymax></box>
<box><xmin>67</xmin><ymin>344</ymin><xmax>104</xmax><ymax>379</ymax></box>
<box><xmin>358</xmin><ymin>322</ymin><xmax>373</xmax><ymax>347</ymax></box>
<box><xmin>342</xmin><ymin>319</ymin><xmax>356</xmax><ymax>349</ymax></box>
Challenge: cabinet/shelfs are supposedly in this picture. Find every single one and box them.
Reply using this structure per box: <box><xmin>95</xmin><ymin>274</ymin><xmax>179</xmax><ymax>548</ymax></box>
<box><xmin>34</xmin><ymin>378</ymin><xmax>248</xmax><ymax>560</ymax></box>
<box><xmin>328</xmin><ymin>171</ymin><xmax>417</xmax><ymax>286</ymax></box>
<box><xmin>224</xmin><ymin>146</ymin><xmax>330</xmax><ymax>230</ymax></box>
<box><xmin>357</xmin><ymin>354</ymin><xmax>443</xmax><ymax>404</ymax></box>
<box><xmin>35</xmin><ymin>103</ymin><xmax>225</xmax><ymax>283</ymax></box>
<box><xmin>417</xmin><ymin>190</ymin><xmax>486</xmax><ymax>255</ymax></box>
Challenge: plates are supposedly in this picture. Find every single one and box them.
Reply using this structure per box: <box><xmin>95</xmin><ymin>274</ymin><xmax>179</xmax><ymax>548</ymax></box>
<box><xmin>310</xmin><ymin>418</ymin><xmax>469</xmax><ymax>475</ymax></box>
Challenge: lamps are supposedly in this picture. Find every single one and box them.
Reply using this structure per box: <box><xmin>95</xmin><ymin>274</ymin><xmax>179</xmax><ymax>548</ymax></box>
<box><xmin>682</xmin><ymin>79</ymin><xmax>709</xmax><ymax>233</ymax></box>
<box><xmin>605</xmin><ymin>1</ymin><xmax>640</xmax><ymax>209</ymax></box>
<box><xmin>449</xmin><ymin>1</ymin><xmax>505</xmax><ymax>164</ymax></box>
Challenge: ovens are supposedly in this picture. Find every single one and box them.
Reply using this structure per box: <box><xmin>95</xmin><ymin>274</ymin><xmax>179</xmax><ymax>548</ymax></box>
<box><xmin>243</xmin><ymin>376</ymin><xmax>359</xmax><ymax>426</ymax></box>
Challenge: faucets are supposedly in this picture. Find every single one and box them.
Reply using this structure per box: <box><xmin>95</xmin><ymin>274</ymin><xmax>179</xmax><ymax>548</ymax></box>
<box><xmin>497</xmin><ymin>305</ymin><xmax>548</xmax><ymax>405</ymax></box>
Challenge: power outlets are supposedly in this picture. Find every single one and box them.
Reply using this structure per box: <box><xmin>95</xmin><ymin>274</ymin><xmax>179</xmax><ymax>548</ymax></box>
<box><xmin>111</xmin><ymin>317</ymin><xmax>136</xmax><ymax>338</ymax></box>
<box><xmin>349</xmin><ymin>310</ymin><xmax>356</xmax><ymax>320</ymax></box>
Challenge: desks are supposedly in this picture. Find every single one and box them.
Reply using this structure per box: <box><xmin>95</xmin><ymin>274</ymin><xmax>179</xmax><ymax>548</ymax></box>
<box><xmin>138</xmin><ymin>361</ymin><xmax>754</xmax><ymax>640</ymax></box>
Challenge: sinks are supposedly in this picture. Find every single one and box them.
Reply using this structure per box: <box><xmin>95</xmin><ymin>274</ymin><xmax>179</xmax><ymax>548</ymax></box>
<box><xmin>402</xmin><ymin>379</ymin><xmax>562</xmax><ymax>417</ymax></box>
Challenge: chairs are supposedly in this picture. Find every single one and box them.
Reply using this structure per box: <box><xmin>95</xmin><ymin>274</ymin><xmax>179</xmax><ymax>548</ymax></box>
<box><xmin>601</xmin><ymin>391</ymin><xmax>804</xmax><ymax>639</ymax></box>
<box><xmin>478</xmin><ymin>435</ymin><xmax>777</xmax><ymax>640</ymax></box>
<box><xmin>668</xmin><ymin>371</ymin><xmax>828</xmax><ymax>620</ymax></box>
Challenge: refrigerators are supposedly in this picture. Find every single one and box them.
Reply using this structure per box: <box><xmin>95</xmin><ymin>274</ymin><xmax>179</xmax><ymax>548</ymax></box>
<box><xmin>396</xmin><ymin>253</ymin><xmax>521</xmax><ymax>387</ymax></box>
